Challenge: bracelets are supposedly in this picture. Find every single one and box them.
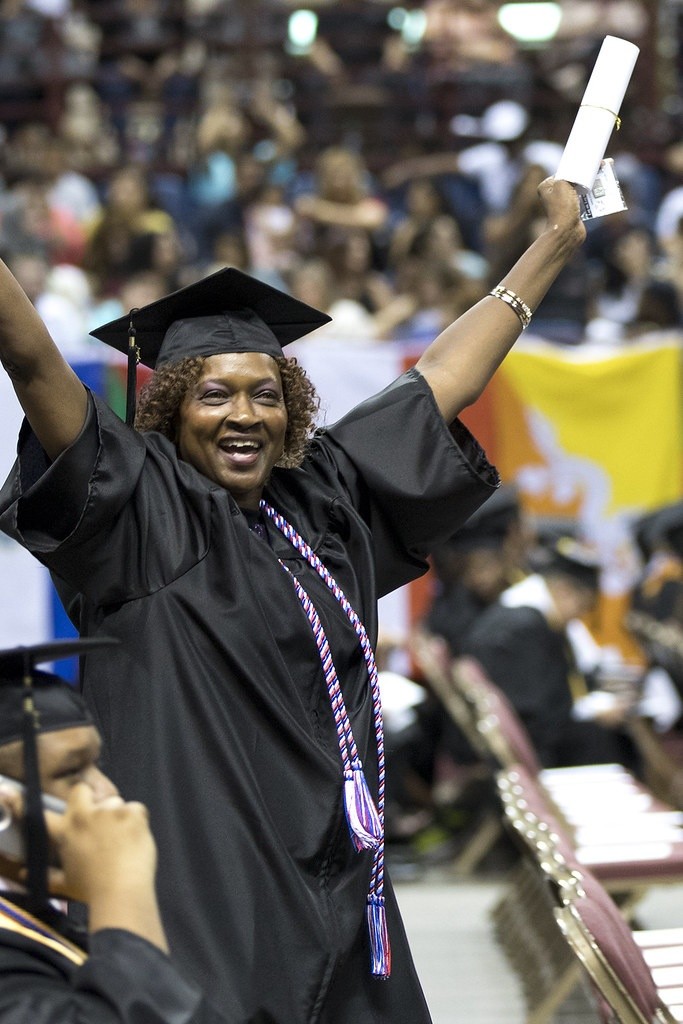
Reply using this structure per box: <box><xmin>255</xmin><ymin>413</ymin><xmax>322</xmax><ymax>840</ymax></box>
<box><xmin>488</xmin><ymin>284</ymin><xmax>532</xmax><ymax>331</ymax></box>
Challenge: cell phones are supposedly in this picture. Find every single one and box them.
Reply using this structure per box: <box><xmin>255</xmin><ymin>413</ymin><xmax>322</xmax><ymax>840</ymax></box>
<box><xmin>0</xmin><ymin>776</ymin><xmax>67</xmax><ymax>869</ymax></box>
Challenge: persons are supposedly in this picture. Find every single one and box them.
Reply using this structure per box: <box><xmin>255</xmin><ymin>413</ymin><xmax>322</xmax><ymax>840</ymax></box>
<box><xmin>0</xmin><ymin>0</ymin><xmax>683</xmax><ymax>1024</ymax></box>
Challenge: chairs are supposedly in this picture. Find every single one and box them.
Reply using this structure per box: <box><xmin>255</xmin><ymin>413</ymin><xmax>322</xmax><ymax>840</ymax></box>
<box><xmin>400</xmin><ymin>611</ymin><xmax>683</xmax><ymax>1024</ymax></box>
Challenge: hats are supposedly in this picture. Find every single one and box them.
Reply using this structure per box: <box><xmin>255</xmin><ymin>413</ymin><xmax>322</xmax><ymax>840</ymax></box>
<box><xmin>1</xmin><ymin>638</ymin><xmax>122</xmax><ymax>923</ymax></box>
<box><xmin>449</xmin><ymin>487</ymin><xmax>521</xmax><ymax>544</ymax></box>
<box><xmin>89</xmin><ymin>266</ymin><xmax>332</xmax><ymax>428</ymax></box>
<box><xmin>534</xmin><ymin>523</ymin><xmax>612</xmax><ymax>588</ymax></box>
